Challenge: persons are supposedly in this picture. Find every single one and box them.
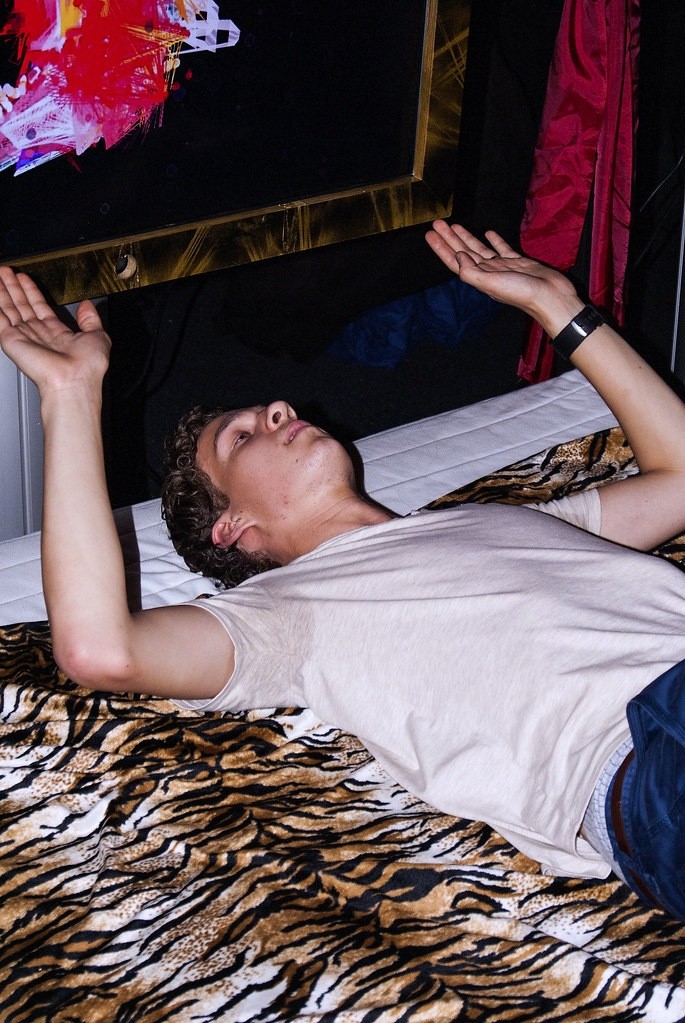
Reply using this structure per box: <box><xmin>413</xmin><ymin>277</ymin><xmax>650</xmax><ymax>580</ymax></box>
<box><xmin>0</xmin><ymin>217</ymin><xmax>684</xmax><ymax>913</ymax></box>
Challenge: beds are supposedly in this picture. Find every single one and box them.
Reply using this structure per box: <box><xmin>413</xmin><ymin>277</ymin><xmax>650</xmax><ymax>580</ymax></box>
<box><xmin>0</xmin><ymin>365</ymin><xmax>685</xmax><ymax>1023</ymax></box>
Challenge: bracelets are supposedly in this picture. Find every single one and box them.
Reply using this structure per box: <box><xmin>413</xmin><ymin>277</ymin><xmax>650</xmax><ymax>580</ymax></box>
<box><xmin>548</xmin><ymin>304</ymin><xmax>606</xmax><ymax>360</ymax></box>
